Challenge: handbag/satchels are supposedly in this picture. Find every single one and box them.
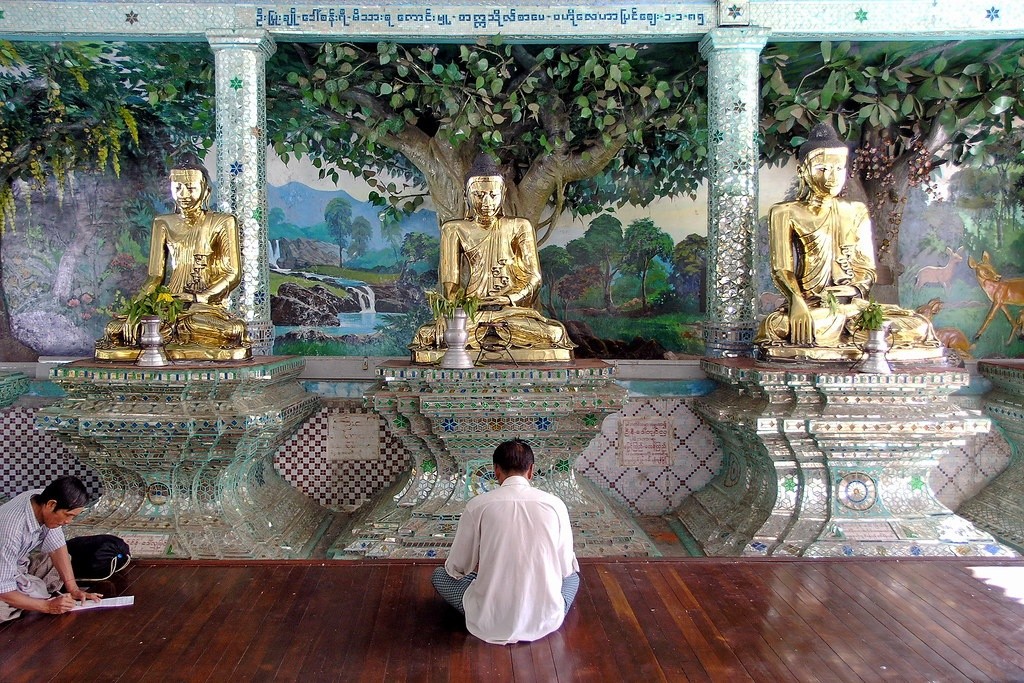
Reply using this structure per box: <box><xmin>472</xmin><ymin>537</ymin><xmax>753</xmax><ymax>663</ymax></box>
<box><xmin>65</xmin><ymin>534</ymin><xmax>131</xmax><ymax>581</ymax></box>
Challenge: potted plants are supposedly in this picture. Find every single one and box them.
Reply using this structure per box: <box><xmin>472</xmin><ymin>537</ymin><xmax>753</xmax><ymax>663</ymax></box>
<box><xmin>117</xmin><ymin>281</ymin><xmax>183</xmax><ymax>366</ymax></box>
<box><xmin>856</xmin><ymin>299</ymin><xmax>895</xmax><ymax>373</ymax></box>
<box><xmin>427</xmin><ymin>280</ymin><xmax>484</xmax><ymax>368</ymax></box>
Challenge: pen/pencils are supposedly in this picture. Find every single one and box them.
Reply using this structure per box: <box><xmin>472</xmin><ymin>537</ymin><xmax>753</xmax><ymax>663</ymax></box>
<box><xmin>56</xmin><ymin>590</ymin><xmax>78</xmax><ymax>605</ymax></box>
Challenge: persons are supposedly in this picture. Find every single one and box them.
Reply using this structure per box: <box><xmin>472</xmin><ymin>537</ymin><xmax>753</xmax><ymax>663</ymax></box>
<box><xmin>759</xmin><ymin>119</ymin><xmax>943</xmax><ymax>347</ymax></box>
<box><xmin>408</xmin><ymin>146</ymin><xmax>581</xmax><ymax>350</ymax></box>
<box><xmin>432</xmin><ymin>441</ymin><xmax>579</xmax><ymax>645</ymax></box>
<box><xmin>0</xmin><ymin>475</ymin><xmax>104</xmax><ymax>624</ymax></box>
<box><xmin>93</xmin><ymin>149</ymin><xmax>253</xmax><ymax>348</ymax></box>
